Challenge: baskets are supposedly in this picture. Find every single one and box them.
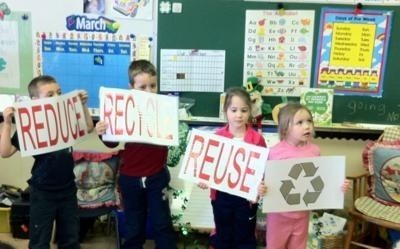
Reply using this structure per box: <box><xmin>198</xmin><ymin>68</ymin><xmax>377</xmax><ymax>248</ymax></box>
<box><xmin>323</xmin><ymin>232</ymin><xmax>362</xmax><ymax>249</ymax></box>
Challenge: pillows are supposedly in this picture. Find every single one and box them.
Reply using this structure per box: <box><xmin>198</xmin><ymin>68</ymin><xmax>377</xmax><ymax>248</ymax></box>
<box><xmin>362</xmin><ymin>139</ymin><xmax>400</xmax><ymax>206</ymax></box>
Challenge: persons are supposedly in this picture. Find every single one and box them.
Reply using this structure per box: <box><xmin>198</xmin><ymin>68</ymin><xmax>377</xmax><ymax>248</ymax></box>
<box><xmin>198</xmin><ymin>87</ymin><xmax>268</xmax><ymax>248</ymax></box>
<box><xmin>0</xmin><ymin>76</ymin><xmax>94</xmax><ymax>249</ymax></box>
<box><xmin>96</xmin><ymin>60</ymin><xmax>177</xmax><ymax>249</ymax></box>
<box><xmin>258</xmin><ymin>103</ymin><xmax>352</xmax><ymax>249</ymax></box>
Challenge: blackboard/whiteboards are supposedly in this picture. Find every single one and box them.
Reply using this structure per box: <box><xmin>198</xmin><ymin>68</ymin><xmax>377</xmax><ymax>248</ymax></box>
<box><xmin>155</xmin><ymin>0</ymin><xmax>400</xmax><ymax>131</ymax></box>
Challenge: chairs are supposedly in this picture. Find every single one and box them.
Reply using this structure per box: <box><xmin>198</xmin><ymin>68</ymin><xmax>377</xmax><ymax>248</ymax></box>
<box><xmin>49</xmin><ymin>150</ymin><xmax>123</xmax><ymax>249</ymax></box>
<box><xmin>345</xmin><ymin>124</ymin><xmax>400</xmax><ymax>249</ymax></box>
